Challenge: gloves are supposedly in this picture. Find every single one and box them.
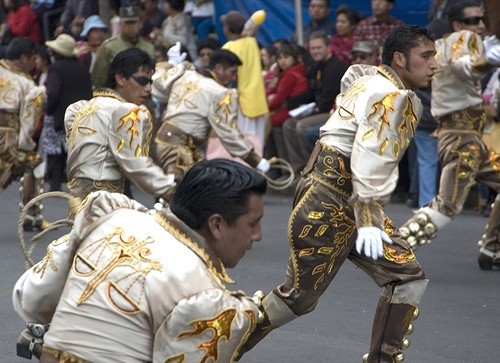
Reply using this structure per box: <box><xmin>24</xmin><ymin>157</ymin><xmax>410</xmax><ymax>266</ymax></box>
<box><xmin>486</xmin><ymin>45</ymin><xmax>500</xmax><ymax>66</ymax></box>
<box><xmin>167</xmin><ymin>42</ymin><xmax>187</xmax><ymax>66</ymax></box>
<box><xmin>354</xmin><ymin>226</ymin><xmax>393</xmax><ymax>259</ymax></box>
<box><xmin>483</xmin><ymin>35</ymin><xmax>498</xmax><ymax>50</ymax></box>
<box><xmin>255</xmin><ymin>159</ymin><xmax>271</xmax><ymax>174</ymax></box>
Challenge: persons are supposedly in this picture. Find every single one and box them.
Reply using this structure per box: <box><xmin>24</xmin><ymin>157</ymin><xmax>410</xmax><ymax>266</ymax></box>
<box><xmin>12</xmin><ymin>158</ymin><xmax>268</xmax><ymax>363</ymax></box>
<box><xmin>0</xmin><ymin>0</ymin><xmax>500</xmax><ymax>363</ymax></box>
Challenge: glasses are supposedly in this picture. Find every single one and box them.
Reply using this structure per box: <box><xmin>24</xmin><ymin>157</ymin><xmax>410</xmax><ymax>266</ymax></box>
<box><xmin>351</xmin><ymin>53</ymin><xmax>371</xmax><ymax>61</ymax></box>
<box><xmin>130</xmin><ymin>75</ymin><xmax>154</xmax><ymax>86</ymax></box>
<box><xmin>457</xmin><ymin>16</ymin><xmax>486</xmax><ymax>25</ymax></box>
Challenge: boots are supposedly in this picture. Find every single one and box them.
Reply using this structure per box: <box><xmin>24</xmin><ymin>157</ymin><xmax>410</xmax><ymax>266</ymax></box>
<box><xmin>478</xmin><ymin>192</ymin><xmax>500</xmax><ymax>271</ymax></box>
<box><xmin>364</xmin><ymin>277</ymin><xmax>429</xmax><ymax>363</ymax></box>
<box><xmin>230</xmin><ymin>290</ymin><xmax>299</xmax><ymax>363</ymax></box>
<box><xmin>19</xmin><ymin>161</ymin><xmax>57</xmax><ymax>231</ymax></box>
<box><xmin>397</xmin><ymin>205</ymin><xmax>452</xmax><ymax>250</ymax></box>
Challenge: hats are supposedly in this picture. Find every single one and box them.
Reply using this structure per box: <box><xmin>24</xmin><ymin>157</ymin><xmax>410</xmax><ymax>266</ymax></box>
<box><xmin>351</xmin><ymin>41</ymin><xmax>376</xmax><ymax>53</ymax></box>
<box><xmin>80</xmin><ymin>15</ymin><xmax>107</xmax><ymax>36</ymax></box>
<box><xmin>119</xmin><ymin>6</ymin><xmax>139</xmax><ymax>21</ymax></box>
<box><xmin>44</xmin><ymin>34</ymin><xmax>74</xmax><ymax>57</ymax></box>
